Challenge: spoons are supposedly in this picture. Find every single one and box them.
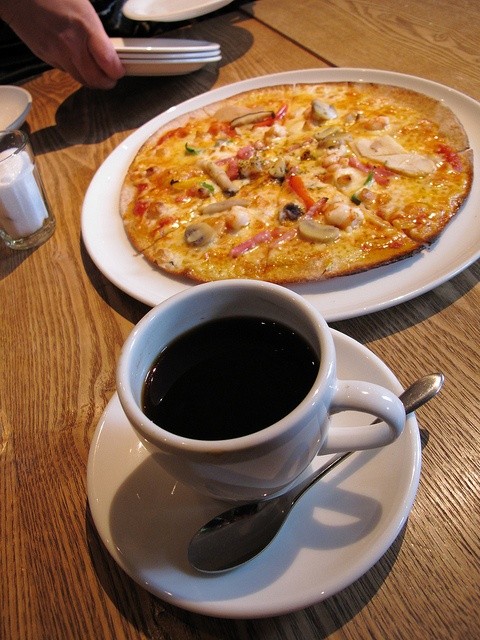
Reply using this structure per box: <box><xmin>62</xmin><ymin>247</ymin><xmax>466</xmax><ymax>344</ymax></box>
<box><xmin>186</xmin><ymin>372</ymin><xmax>444</xmax><ymax>576</ymax></box>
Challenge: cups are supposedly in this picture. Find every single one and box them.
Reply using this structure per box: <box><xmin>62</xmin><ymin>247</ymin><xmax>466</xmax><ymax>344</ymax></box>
<box><xmin>0</xmin><ymin>131</ymin><xmax>54</xmax><ymax>251</ymax></box>
<box><xmin>115</xmin><ymin>278</ymin><xmax>405</xmax><ymax>505</ymax></box>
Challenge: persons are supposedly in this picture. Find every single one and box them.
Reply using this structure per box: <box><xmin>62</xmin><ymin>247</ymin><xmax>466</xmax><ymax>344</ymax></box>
<box><xmin>0</xmin><ymin>0</ymin><xmax>141</xmax><ymax>97</ymax></box>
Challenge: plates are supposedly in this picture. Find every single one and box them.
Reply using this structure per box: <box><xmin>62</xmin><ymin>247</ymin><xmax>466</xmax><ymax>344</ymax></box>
<box><xmin>0</xmin><ymin>85</ymin><xmax>31</xmax><ymax>137</ymax></box>
<box><xmin>87</xmin><ymin>327</ymin><xmax>422</xmax><ymax>618</ymax></box>
<box><xmin>121</xmin><ymin>1</ymin><xmax>233</xmax><ymax>23</ymax></box>
<box><xmin>109</xmin><ymin>37</ymin><xmax>222</xmax><ymax>76</ymax></box>
<box><xmin>81</xmin><ymin>67</ymin><xmax>480</xmax><ymax>322</ymax></box>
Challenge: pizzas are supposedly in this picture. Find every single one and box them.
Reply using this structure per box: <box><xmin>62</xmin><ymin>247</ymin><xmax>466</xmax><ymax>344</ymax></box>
<box><xmin>117</xmin><ymin>81</ymin><xmax>475</xmax><ymax>286</ymax></box>
